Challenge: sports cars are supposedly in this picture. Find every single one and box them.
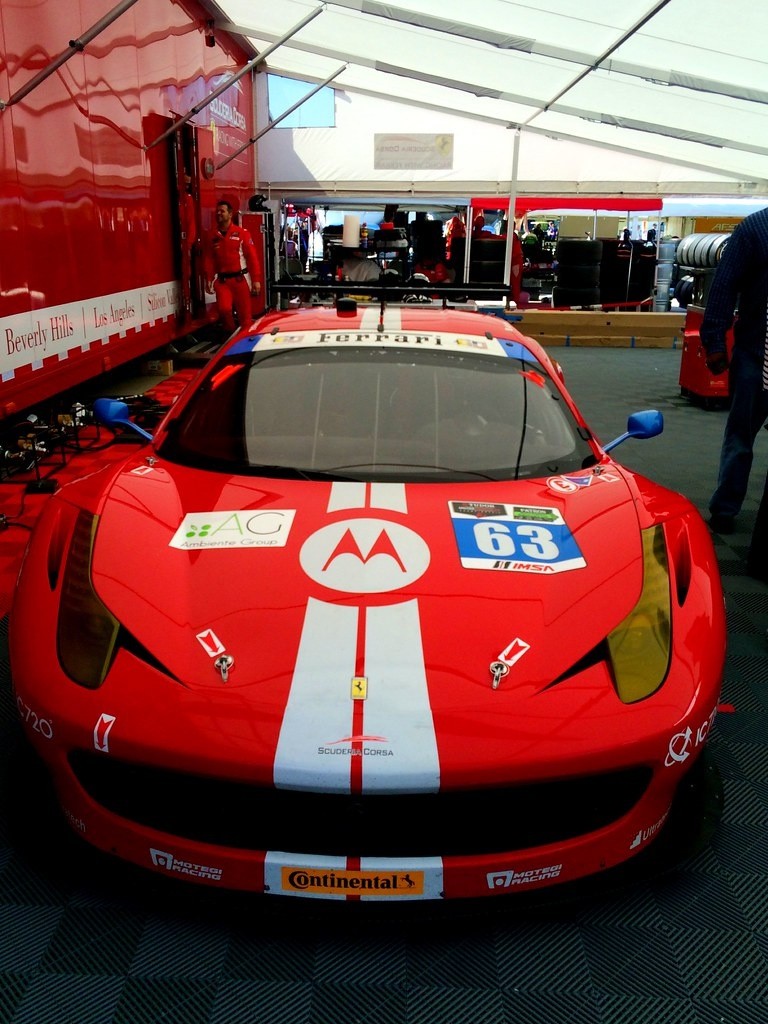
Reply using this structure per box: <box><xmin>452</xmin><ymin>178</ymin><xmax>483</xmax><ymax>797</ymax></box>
<box><xmin>7</xmin><ymin>285</ymin><xmax>738</xmax><ymax>904</ymax></box>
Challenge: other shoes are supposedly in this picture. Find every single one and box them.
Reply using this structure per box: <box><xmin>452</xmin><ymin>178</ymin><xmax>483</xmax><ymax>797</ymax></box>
<box><xmin>705</xmin><ymin>514</ymin><xmax>736</xmax><ymax>534</ymax></box>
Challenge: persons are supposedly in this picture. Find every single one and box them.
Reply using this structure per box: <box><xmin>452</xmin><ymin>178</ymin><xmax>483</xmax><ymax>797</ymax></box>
<box><xmin>549</xmin><ymin>224</ymin><xmax>558</xmax><ymax>241</ymax></box>
<box><xmin>472</xmin><ymin>216</ymin><xmax>491</xmax><ymax>239</ymax></box>
<box><xmin>310</xmin><ymin>214</ymin><xmax>320</xmax><ymax>231</ymax></box>
<box><xmin>504</xmin><ymin>220</ymin><xmax>524</xmax><ymax>304</ymax></box>
<box><xmin>410</xmin><ymin>212</ymin><xmax>427</xmax><ymax>241</ymax></box>
<box><xmin>700</xmin><ymin>208</ymin><xmax>768</xmax><ymax>586</ymax></box>
<box><xmin>203</xmin><ymin>200</ymin><xmax>262</xmax><ymax>331</ymax></box>
<box><xmin>533</xmin><ymin>224</ymin><xmax>544</xmax><ymax>242</ymax></box>
<box><xmin>494</xmin><ymin>208</ymin><xmax>507</xmax><ymax>236</ymax></box>
<box><xmin>523</xmin><ymin>234</ymin><xmax>542</xmax><ymax>262</ymax></box>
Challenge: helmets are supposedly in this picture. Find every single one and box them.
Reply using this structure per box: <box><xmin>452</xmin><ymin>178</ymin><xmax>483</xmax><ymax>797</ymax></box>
<box><xmin>248</xmin><ymin>194</ymin><xmax>271</xmax><ymax>212</ymax></box>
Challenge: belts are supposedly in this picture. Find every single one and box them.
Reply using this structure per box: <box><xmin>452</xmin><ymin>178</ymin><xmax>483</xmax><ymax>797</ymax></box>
<box><xmin>218</xmin><ymin>268</ymin><xmax>249</xmax><ymax>279</ymax></box>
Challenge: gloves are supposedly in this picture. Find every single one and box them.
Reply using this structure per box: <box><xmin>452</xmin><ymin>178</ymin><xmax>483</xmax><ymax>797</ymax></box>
<box><xmin>704</xmin><ymin>343</ymin><xmax>730</xmax><ymax>375</ymax></box>
